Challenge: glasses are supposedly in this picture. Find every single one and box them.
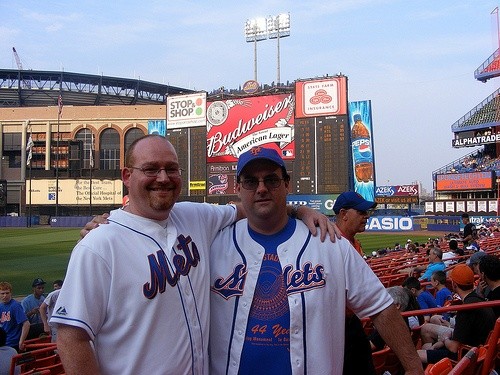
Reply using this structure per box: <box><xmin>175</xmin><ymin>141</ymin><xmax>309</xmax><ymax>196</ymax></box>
<box><xmin>237</xmin><ymin>175</ymin><xmax>284</xmax><ymax>190</ymax></box>
<box><xmin>126</xmin><ymin>166</ymin><xmax>184</xmax><ymax>179</ymax></box>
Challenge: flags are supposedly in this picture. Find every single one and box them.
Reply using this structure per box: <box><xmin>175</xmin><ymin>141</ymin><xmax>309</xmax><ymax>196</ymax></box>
<box><xmin>58</xmin><ymin>94</ymin><xmax>63</xmax><ymax>118</ymax></box>
<box><xmin>27</xmin><ymin>147</ymin><xmax>32</xmax><ymax>165</ymax></box>
<box><xmin>26</xmin><ymin>131</ymin><xmax>34</xmax><ymax>150</ymax></box>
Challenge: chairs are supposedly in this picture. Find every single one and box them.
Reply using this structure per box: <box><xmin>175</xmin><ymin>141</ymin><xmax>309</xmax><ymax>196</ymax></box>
<box><xmin>9</xmin><ymin>230</ymin><xmax>500</xmax><ymax>375</ymax></box>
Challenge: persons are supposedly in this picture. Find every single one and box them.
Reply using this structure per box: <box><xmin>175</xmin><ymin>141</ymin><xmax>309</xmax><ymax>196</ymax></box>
<box><xmin>49</xmin><ymin>135</ymin><xmax>341</xmax><ymax>375</ymax></box>
<box><xmin>446</xmin><ymin>149</ymin><xmax>500</xmax><ymax>173</ymax></box>
<box><xmin>78</xmin><ymin>146</ymin><xmax>424</xmax><ymax>375</ymax></box>
<box><xmin>356</xmin><ymin>213</ymin><xmax>500</xmax><ymax>375</ymax></box>
<box><xmin>332</xmin><ymin>192</ymin><xmax>377</xmax><ymax>375</ymax></box>
<box><xmin>20</xmin><ymin>277</ymin><xmax>63</xmax><ymax>343</ymax></box>
<box><xmin>0</xmin><ymin>282</ymin><xmax>31</xmax><ymax>353</ymax></box>
<box><xmin>0</xmin><ymin>326</ymin><xmax>21</xmax><ymax>375</ymax></box>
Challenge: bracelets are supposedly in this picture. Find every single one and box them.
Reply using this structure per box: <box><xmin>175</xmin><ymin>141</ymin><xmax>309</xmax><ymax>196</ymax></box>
<box><xmin>291</xmin><ymin>204</ymin><xmax>302</xmax><ymax>219</ymax></box>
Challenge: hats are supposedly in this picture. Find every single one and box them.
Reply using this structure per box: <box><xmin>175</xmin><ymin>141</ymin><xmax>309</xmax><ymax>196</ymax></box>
<box><xmin>333</xmin><ymin>191</ymin><xmax>377</xmax><ymax>214</ymax></box>
<box><xmin>236</xmin><ymin>146</ymin><xmax>287</xmax><ymax>175</ymax></box>
<box><xmin>466</xmin><ymin>244</ymin><xmax>480</xmax><ymax>252</ymax></box>
<box><xmin>448</xmin><ymin>264</ymin><xmax>474</xmax><ymax>285</ymax></box>
<box><xmin>468</xmin><ymin>252</ymin><xmax>489</xmax><ymax>267</ymax></box>
<box><xmin>453</xmin><ymin>249</ymin><xmax>464</xmax><ymax>256</ymax></box>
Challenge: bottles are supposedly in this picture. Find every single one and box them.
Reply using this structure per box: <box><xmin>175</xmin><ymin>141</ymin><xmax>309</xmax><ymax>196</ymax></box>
<box><xmin>351</xmin><ymin>114</ymin><xmax>373</xmax><ymax>182</ymax></box>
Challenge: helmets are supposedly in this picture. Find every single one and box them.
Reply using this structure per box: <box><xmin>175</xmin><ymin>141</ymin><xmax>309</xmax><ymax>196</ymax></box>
<box><xmin>32</xmin><ymin>278</ymin><xmax>46</xmax><ymax>287</ymax></box>
